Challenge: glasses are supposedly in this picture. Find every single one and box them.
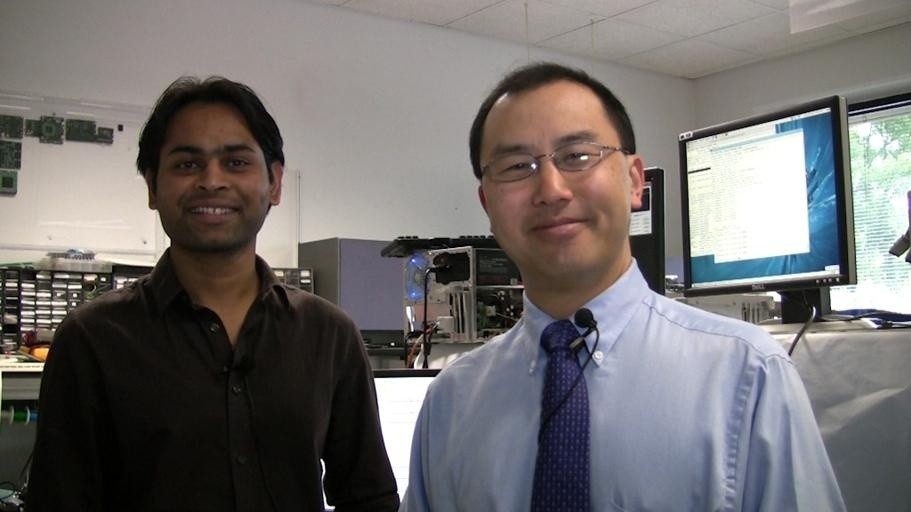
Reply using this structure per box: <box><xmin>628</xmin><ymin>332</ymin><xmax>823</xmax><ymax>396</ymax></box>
<box><xmin>480</xmin><ymin>140</ymin><xmax>624</xmax><ymax>184</ymax></box>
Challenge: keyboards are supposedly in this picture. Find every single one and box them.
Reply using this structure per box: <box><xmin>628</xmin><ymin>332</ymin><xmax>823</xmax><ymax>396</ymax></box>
<box><xmin>380</xmin><ymin>234</ymin><xmax>499</xmax><ymax>258</ymax></box>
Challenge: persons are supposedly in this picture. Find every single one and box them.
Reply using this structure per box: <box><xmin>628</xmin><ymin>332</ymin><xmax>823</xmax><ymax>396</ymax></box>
<box><xmin>25</xmin><ymin>73</ymin><xmax>402</xmax><ymax>511</ymax></box>
<box><xmin>393</xmin><ymin>61</ymin><xmax>852</xmax><ymax>511</ymax></box>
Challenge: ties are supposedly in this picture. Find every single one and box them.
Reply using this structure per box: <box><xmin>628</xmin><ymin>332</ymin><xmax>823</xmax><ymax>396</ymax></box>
<box><xmin>528</xmin><ymin>319</ymin><xmax>591</xmax><ymax>512</ymax></box>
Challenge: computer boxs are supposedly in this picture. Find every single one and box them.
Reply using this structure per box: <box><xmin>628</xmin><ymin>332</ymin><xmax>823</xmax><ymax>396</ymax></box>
<box><xmin>400</xmin><ymin>247</ymin><xmax>526</xmax><ymax>343</ymax></box>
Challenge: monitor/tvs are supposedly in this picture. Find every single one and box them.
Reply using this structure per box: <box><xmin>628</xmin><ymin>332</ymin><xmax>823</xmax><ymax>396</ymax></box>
<box><xmin>679</xmin><ymin>94</ymin><xmax>877</xmax><ymax>333</ymax></box>
